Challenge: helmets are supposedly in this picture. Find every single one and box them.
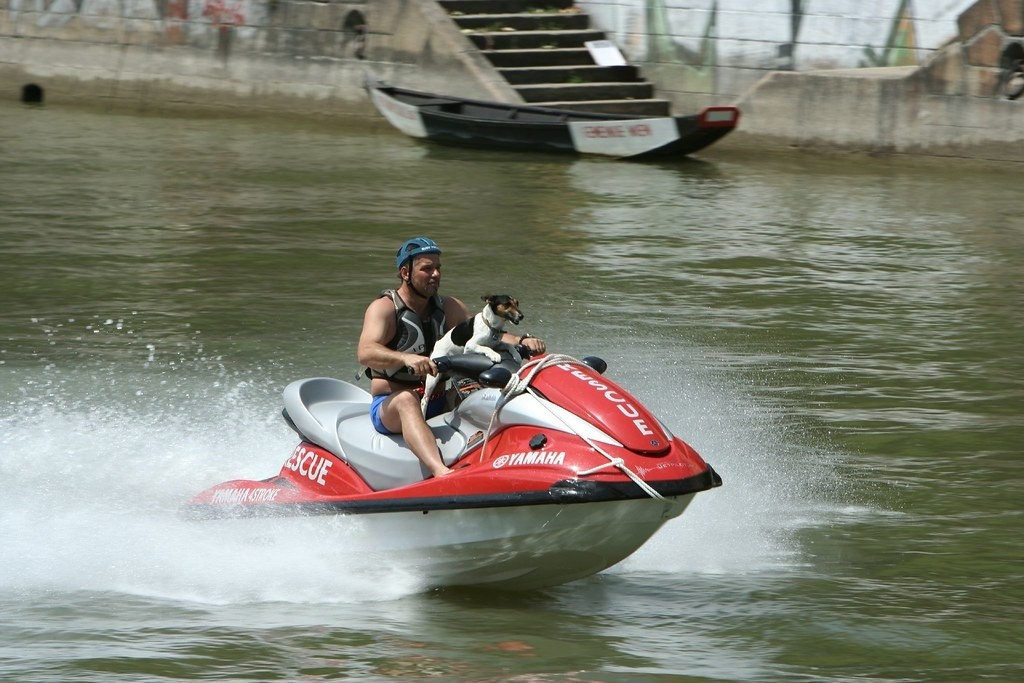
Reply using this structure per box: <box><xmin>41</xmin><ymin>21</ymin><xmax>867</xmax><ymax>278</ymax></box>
<box><xmin>396</xmin><ymin>236</ymin><xmax>440</xmax><ymax>270</ymax></box>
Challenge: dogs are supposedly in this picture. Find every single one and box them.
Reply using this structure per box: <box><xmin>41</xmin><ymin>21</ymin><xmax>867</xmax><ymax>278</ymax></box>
<box><xmin>420</xmin><ymin>294</ymin><xmax>524</xmax><ymax>421</ymax></box>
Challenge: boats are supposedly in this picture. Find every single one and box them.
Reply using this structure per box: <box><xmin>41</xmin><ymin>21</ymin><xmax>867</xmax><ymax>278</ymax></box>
<box><xmin>358</xmin><ymin>78</ymin><xmax>743</xmax><ymax>164</ymax></box>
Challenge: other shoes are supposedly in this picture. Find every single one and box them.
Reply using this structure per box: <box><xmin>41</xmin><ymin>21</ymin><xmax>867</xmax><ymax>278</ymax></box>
<box><xmin>455</xmin><ymin>463</ymin><xmax>470</xmax><ymax>470</ymax></box>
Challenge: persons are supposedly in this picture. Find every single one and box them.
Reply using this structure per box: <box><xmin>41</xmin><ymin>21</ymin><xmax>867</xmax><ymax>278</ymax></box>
<box><xmin>358</xmin><ymin>237</ymin><xmax>546</xmax><ymax>478</ymax></box>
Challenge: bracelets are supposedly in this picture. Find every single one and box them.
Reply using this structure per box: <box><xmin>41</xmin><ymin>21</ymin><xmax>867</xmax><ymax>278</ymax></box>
<box><xmin>519</xmin><ymin>333</ymin><xmax>534</xmax><ymax>344</ymax></box>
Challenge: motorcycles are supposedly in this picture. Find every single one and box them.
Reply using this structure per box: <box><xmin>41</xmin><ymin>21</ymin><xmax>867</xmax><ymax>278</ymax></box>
<box><xmin>172</xmin><ymin>343</ymin><xmax>725</xmax><ymax>593</ymax></box>
